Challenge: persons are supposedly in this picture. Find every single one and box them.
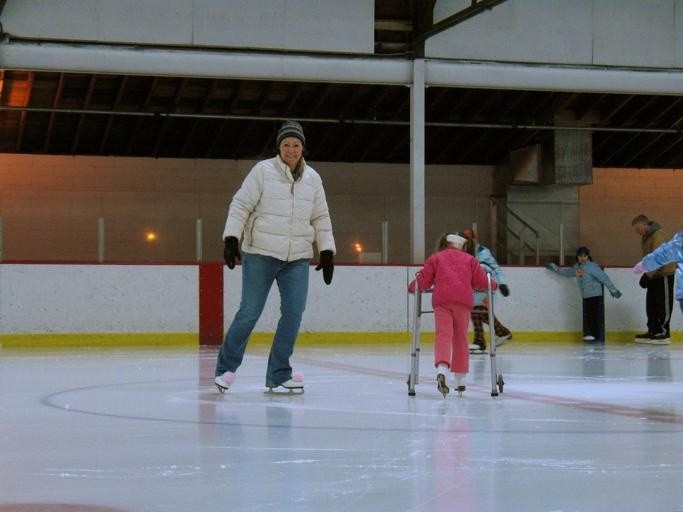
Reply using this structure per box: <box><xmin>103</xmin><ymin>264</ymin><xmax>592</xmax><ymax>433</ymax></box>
<box><xmin>210</xmin><ymin>119</ymin><xmax>337</xmax><ymax>390</ymax></box>
<box><xmin>406</xmin><ymin>230</ymin><xmax>498</xmax><ymax>394</ymax></box>
<box><xmin>632</xmin><ymin>230</ymin><xmax>682</xmax><ymax>312</ymax></box>
<box><xmin>456</xmin><ymin>227</ymin><xmax>512</xmax><ymax>351</ymax></box>
<box><xmin>631</xmin><ymin>214</ymin><xmax>676</xmax><ymax>341</ymax></box>
<box><xmin>544</xmin><ymin>245</ymin><xmax>622</xmax><ymax>342</ymax></box>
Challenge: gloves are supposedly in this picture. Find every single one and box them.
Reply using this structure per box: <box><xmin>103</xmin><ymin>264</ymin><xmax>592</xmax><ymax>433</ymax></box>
<box><xmin>611</xmin><ymin>291</ymin><xmax>621</xmax><ymax>298</ymax></box>
<box><xmin>223</xmin><ymin>236</ymin><xmax>240</xmax><ymax>269</ymax></box>
<box><xmin>632</xmin><ymin>262</ymin><xmax>643</xmax><ymax>273</ymax></box>
<box><xmin>639</xmin><ymin>274</ymin><xmax>648</xmax><ymax>288</ymax></box>
<box><xmin>498</xmin><ymin>284</ymin><xmax>508</xmax><ymax>296</ymax></box>
<box><xmin>315</xmin><ymin>251</ymin><xmax>332</xmax><ymax>284</ymax></box>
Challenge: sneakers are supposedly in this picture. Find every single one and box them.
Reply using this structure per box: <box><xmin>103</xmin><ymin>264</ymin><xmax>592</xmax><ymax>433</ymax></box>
<box><xmin>635</xmin><ymin>328</ymin><xmax>670</xmax><ymax>340</ymax></box>
<box><xmin>280</xmin><ymin>380</ymin><xmax>303</xmax><ymax>388</ymax></box>
<box><xmin>454</xmin><ymin>375</ymin><xmax>464</xmax><ymax>391</ymax></box>
<box><xmin>468</xmin><ymin>344</ymin><xmax>480</xmax><ymax>350</ymax></box>
<box><xmin>436</xmin><ymin>363</ymin><xmax>449</xmax><ymax>394</ymax></box>
<box><xmin>584</xmin><ymin>336</ymin><xmax>596</xmax><ymax>340</ymax></box>
<box><xmin>495</xmin><ymin>334</ymin><xmax>512</xmax><ymax>346</ymax></box>
<box><xmin>214</xmin><ymin>376</ymin><xmax>229</xmax><ymax>388</ymax></box>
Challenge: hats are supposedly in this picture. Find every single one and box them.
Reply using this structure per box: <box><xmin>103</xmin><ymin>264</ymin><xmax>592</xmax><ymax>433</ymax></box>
<box><xmin>275</xmin><ymin>121</ymin><xmax>306</xmax><ymax>148</ymax></box>
